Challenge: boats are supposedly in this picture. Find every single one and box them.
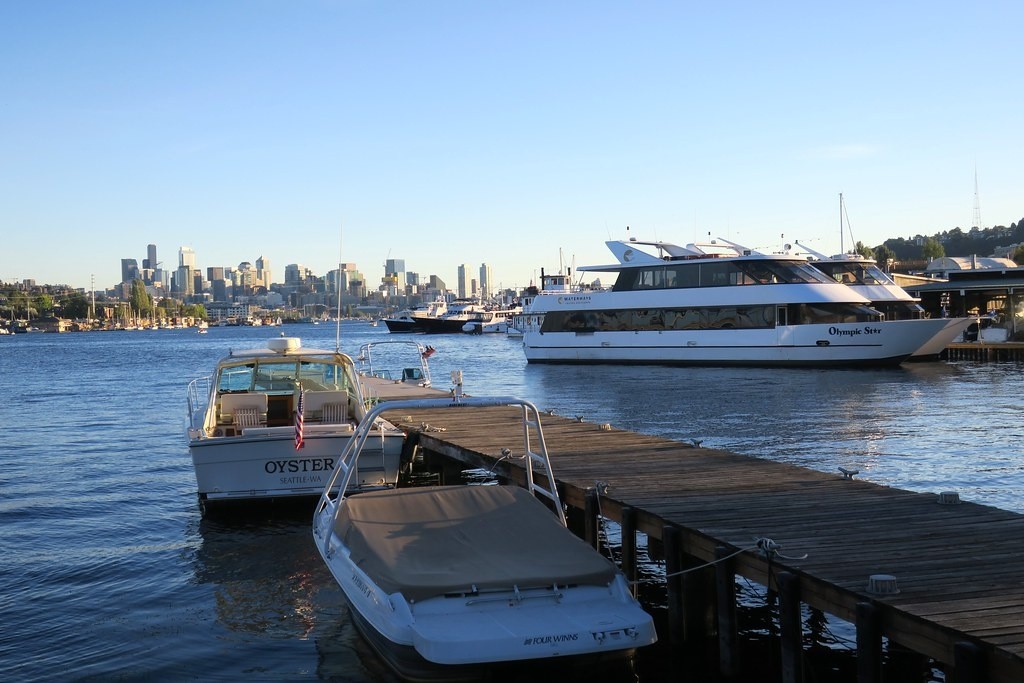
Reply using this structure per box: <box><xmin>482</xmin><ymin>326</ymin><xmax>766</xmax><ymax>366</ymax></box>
<box><xmin>380</xmin><ymin>317</ymin><xmax>387</xmax><ymax>321</ymax></box>
<box><xmin>197</xmin><ymin>329</ymin><xmax>209</xmax><ymax>334</ymax></box>
<box><xmin>369</xmin><ymin>320</ymin><xmax>379</xmax><ymax>327</ymax></box>
<box><xmin>312</xmin><ymin>372</ymin><xmax>659</xmax><ymax>683</ymax></box>
<box><xmin>184</xmin><ymin>331</ymin><xmax>407</xmax><ymax>518</ymax></box>
<box><xmin>383</xmin><ymin>192</ymin><xmax>1024</xmax><ymax>369</ymax></box>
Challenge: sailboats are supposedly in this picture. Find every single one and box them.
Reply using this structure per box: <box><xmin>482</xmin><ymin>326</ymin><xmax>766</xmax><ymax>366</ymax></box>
<box><xmin>276</xmin><ymin>317</ymin><xmax>284</xmax><ymax>326</ymax></box>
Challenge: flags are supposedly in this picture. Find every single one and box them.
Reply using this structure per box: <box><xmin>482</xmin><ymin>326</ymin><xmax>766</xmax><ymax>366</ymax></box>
<box><xmin>294</xmin><ymin>385</ymin><xmax>304</xmax><ymax>452</ymax></box>
